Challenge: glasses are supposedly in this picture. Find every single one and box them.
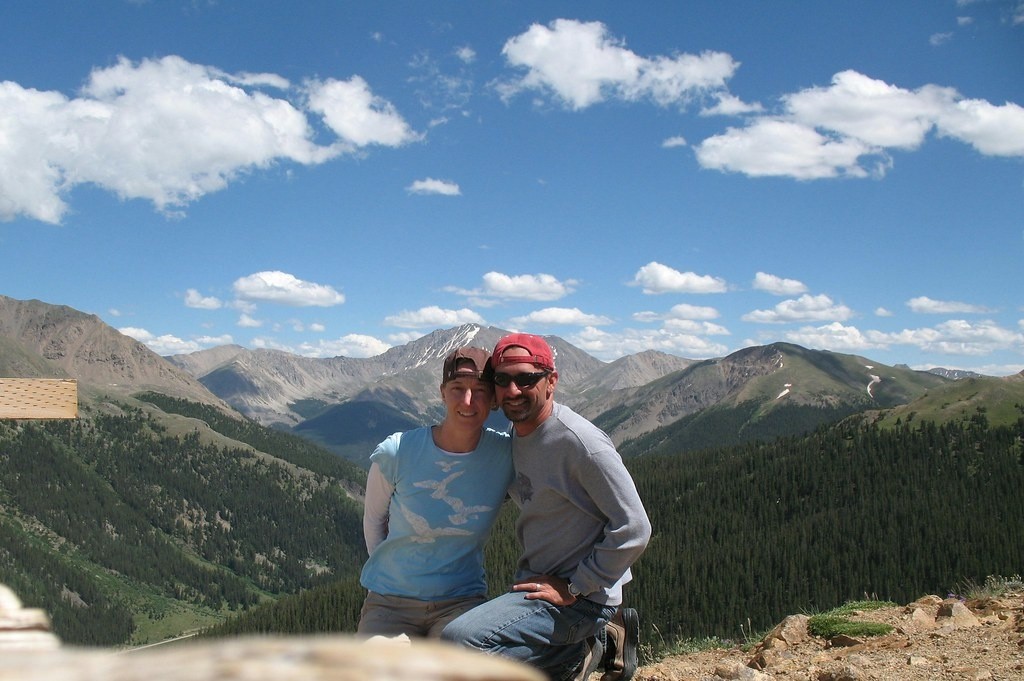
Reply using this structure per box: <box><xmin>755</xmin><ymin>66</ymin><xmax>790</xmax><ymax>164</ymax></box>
<box><xmin>492</xmin><ymin>371</ymin><xmax>552</xmax><ymax>387</ymax></box>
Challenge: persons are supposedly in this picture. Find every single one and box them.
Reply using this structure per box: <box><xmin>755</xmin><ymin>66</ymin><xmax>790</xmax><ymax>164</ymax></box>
<box><xmin>438</xmin><ymin>333</ymin><xmax>652</xmax><ymax>680</ymax></box>
<box><xmin>355</xmin><ymin>347</ymin><xmax>511</xmax><ymax>641</ymax></box>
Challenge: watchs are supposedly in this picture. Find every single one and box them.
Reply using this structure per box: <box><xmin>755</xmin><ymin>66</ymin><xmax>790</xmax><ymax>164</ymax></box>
<box><xmin>567</xmin><ymin>582</ymin><xmax>581</xmax><ymax>599</ymax></box>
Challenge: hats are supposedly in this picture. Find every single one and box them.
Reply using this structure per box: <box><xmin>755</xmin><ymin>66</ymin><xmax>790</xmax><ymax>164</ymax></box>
<box><xmin>443</xmin><ymin>346</ymin><xmax>492</xmax><ymax>384</ymax></box>
<box><xmin>491</xmin><ymin>333</ymin><xmax>554</xmax><ymax>371</ymax></box>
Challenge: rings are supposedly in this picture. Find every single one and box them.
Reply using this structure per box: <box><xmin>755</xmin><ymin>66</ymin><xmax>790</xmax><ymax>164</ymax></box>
<box><xmin>536</xmin><ymin>584</ymin><xmax>540</xmax><ymax>591</ymax></box>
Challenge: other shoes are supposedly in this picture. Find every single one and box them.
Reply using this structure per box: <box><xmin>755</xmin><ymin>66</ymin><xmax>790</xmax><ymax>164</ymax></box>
<box><xmin>599</xmin><ymin>608</ymin><xmax>639</xmax><ymax>681</ymax></box>
<box><xmin>563</xmin><ymin>635</ymin><xmax>603</xmax><ymax>681</ymax></box>
